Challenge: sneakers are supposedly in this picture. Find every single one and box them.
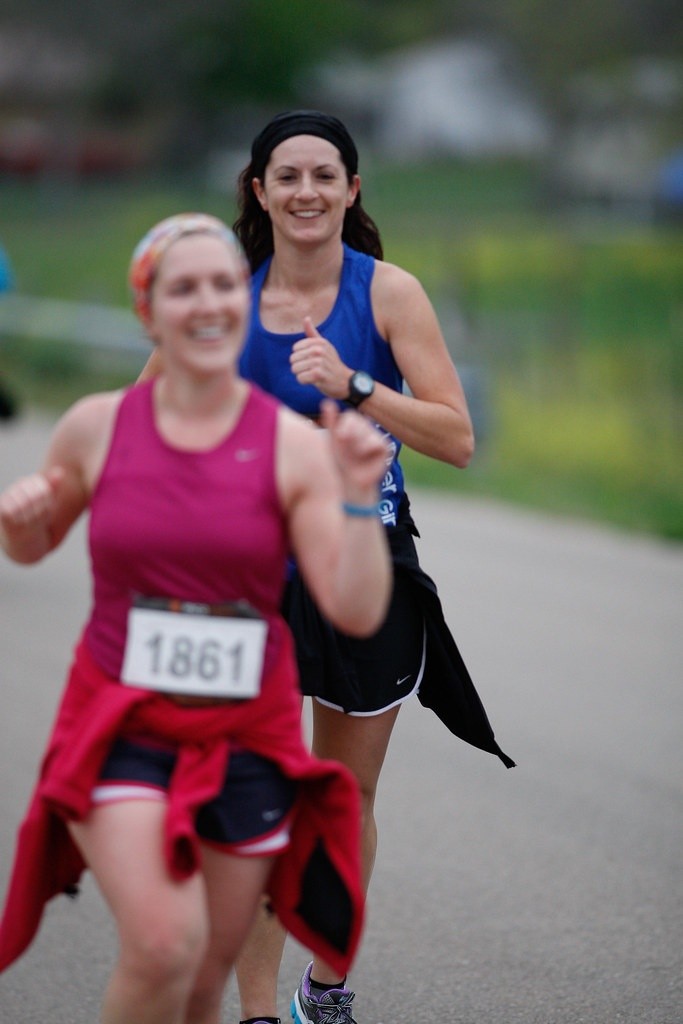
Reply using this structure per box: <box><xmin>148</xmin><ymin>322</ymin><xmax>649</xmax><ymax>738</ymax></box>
<box><xmin>293</xmin><ymin>961</ymin><xmax>358</xmax><ymax>1024</ymax></box>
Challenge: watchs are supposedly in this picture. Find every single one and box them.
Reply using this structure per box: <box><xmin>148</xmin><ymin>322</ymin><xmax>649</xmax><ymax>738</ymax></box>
<box><xmin>342</xmin><ymin>370</ymin><xmax>375</xmax><ymax>408</ymax></box>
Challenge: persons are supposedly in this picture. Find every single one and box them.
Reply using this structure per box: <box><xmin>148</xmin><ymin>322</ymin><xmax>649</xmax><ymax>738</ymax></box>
<box><xmin>0</xmin><ymin>211</ymin><xmax>393</xmax><ymax>1024</ymax></box>
<box><xmin>136</xmin><ymin>110</ymin><xmax>475</xmax><ymax>1024</ymax></box>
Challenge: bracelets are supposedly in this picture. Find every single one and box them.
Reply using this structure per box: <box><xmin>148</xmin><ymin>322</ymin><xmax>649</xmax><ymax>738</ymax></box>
<box><xmin>343</xmin><ymin>501</ymin><xmax>378</xmax><ymax>517</ymax></box>
